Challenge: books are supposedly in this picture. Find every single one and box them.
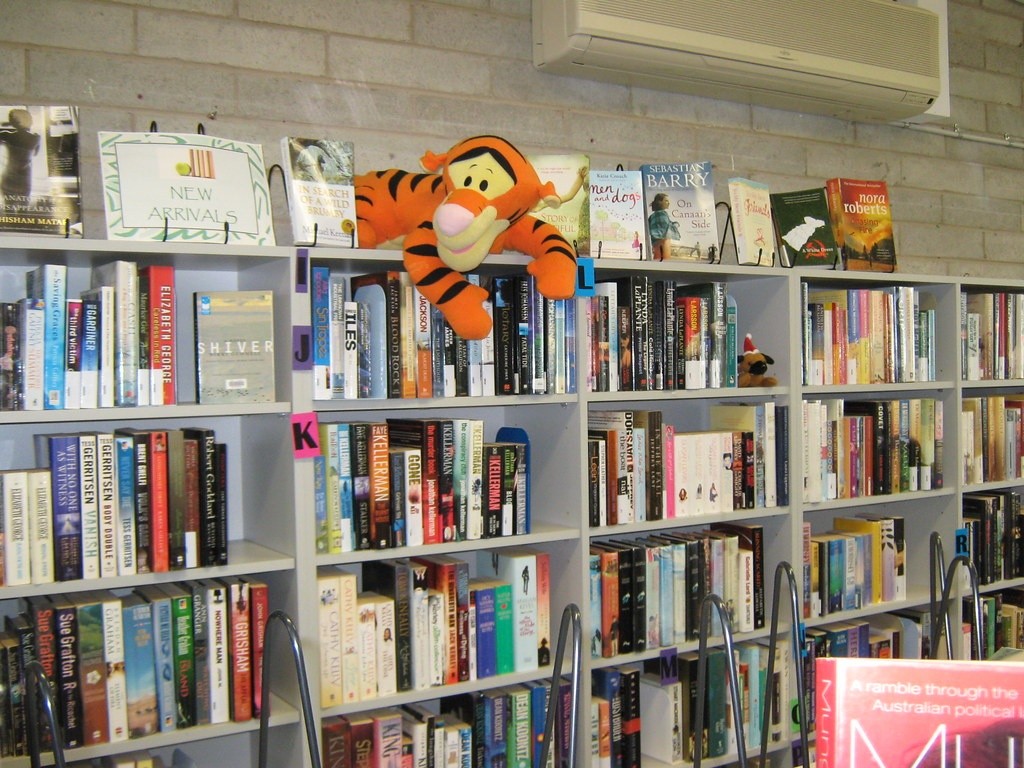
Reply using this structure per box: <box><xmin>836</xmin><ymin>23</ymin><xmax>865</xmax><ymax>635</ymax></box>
<box><xmin>728</xmin><ymin>177</ymin><xmax>775</xmax><ymax>266</ymax></box>
<box><xmin>803</xmin><ymin>283</ymin><xmax>936</xmax><ymax>386</ymax></box>
<box><xmin>960</xmin><ymin>292</ymin><xmax>1023</xmax><ymax>381</ymax></box>
<box><xmin>283</xmin><ymin>137</ymin><xmax>358</xmax><ymax>247</ymax></box>
<box><xmin>524</xmin><ymin>154</ymin><xmax>591</xmax><ymax>258</ymax></box>
<box><xmin>313</xmin><ymin>268</ymin><xmax>576</xmax><ymax>400</ymax></box>
<box><xmin>587</xmin><ymin>276</ymin><xmax>738</xmax><ymax>393</ymax></box>
<box><xmin>0</xmin><ymin>574</ymin><xmax>270</xmax><ymax>767</ymax></box>
<box><xmin>193</xmin><ymin>291</ymin><xmax>275</xmax><ymax>404</ymax></box>
<box><xmin>589</xmin><ymin>170</ymin><xmax>648</xmax><ymax>261</ymax></box>
<box><xmin>639</xmin><ymin>160</ymin><xmax>721</xmax><ymax>264</ymax></box>
<box><xmin>0</xmin><ymin>104</ymin><xmax>84</xmax><ymax>236</ymax></box>
<box><xmin>0</xmin><ymin>427</ymin><xmax>228</xmax><ymax>587</ymax></box>
<box><xmin>770</xmin><ymin>187</ymin><xmax>841</xmax><ymax>269</ymax></box>
<box><xmin>0</xmin><ymin>262</ymin><xmax>176</xmax><ymax>412</ymax></box>
<box><xmin>826</xmin><ymin>178</ymin><xmax>899</xmax><ymax>273</ymax></box>
<box><xmin>322</xmin><ymin>394</ymin><xmax>1024</xmax><ymax>768</ymax></box>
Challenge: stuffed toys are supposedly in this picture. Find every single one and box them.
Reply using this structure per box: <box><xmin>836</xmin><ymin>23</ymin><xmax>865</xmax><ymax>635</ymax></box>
<box><xmin>738</xmin><ymin>334</ymin><xmax>777</xmax><ymax>388</ymax></box>
<box><xmin>356</xmin><ymin>135</ymin><xmax>577</xmax><ymax>341</ymax></box>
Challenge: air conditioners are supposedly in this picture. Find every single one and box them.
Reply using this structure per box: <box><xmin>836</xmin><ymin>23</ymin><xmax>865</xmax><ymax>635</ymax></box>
<box><xmin>529</xmin><ymin>0</ymin><xmax>940</xmax><ymax>127</ymax></box>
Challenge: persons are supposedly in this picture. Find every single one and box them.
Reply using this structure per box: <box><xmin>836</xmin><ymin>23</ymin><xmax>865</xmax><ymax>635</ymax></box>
<box><xmin>0</xmin><ymin>109</ymin><xmax>40</xmax><ymax>212</ymax></box>
<box><xmin>649</xmin><ymin>192</ymin><xmax>681</xmax><ymax>260</ymax></box>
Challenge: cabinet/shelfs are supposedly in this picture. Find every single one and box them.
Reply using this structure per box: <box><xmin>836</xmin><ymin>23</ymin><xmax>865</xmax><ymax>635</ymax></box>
<box><xmin>0</xmin><ymin>232</ymin><xmax>1024</xmax><ymax>768</ymax></box>
